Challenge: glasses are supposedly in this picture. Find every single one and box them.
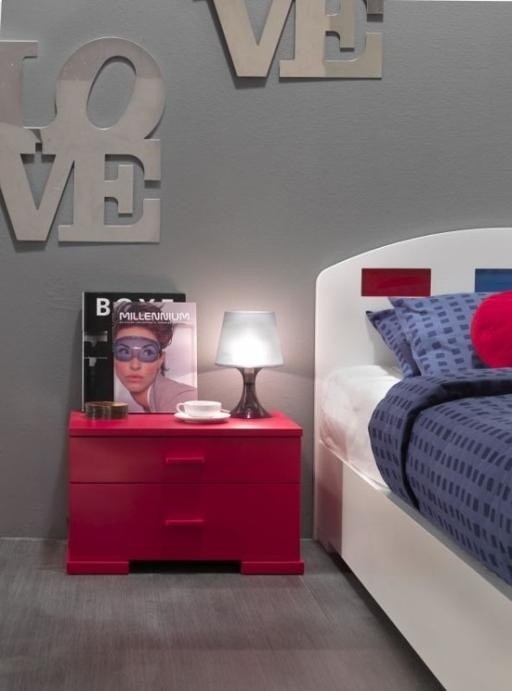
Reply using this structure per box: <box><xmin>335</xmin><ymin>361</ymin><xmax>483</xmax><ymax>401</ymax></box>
<box><xmin>113</xmin><ymin>336</ymin><xmax>163</xmax><ymax>363</ymax></box>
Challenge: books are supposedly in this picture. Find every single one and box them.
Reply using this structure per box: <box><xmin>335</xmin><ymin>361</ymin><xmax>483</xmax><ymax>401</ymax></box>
<box><xmin>81</xmin><ymin>292</ymin><xmax>198</xmax><ymax>413</ymax></box>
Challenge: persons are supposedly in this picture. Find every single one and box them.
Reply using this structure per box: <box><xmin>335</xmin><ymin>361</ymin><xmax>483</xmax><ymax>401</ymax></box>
<box><xmin>114</xmin><ymin>301</ymin><xmax>197</xmax><ymax>412</ymax></box>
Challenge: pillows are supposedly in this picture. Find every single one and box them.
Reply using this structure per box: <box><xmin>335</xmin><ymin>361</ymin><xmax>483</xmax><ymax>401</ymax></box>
<box><xmin>390</xmin><ymin>287</ymin><xmax>512</xmax><ymax>375</ymax></box>
<box><xmin>474</xmin><ymin>292</ymin><xmax>511</xmax><ymax>366</ymax></box>
<box><xmin>362</xmin><ymin>299</ymin><xmax>420</xmax><ymax>381</ymax></box>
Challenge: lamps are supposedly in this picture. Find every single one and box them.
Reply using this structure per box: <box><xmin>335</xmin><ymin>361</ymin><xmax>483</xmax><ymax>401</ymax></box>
<box><xmin>214</xmin><ymin>307</ymin><xmax>287</xmax><ymax>419</ymax></box>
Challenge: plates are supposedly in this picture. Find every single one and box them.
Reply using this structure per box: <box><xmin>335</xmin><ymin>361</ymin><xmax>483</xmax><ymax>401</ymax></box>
<box><xmin>173</xmin><ymin>412</ymin><xmax>232</xmax><ymax>422</ymax></box>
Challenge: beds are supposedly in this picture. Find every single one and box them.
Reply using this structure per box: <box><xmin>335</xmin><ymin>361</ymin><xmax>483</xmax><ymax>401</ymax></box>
<box><xmin>313</xmin><ymin>226</ymin><xmax>512</xmax><ymax>686</ymax></box>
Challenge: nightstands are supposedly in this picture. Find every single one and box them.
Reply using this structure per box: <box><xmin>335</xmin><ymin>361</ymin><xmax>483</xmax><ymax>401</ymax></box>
<box><xmin>65</xmin><ymin>410</ymin><xmax>305</xmax><ymax>575</ymax></box>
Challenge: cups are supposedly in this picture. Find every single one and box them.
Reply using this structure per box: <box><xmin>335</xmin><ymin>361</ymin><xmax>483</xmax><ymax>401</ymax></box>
<box><xmin>177</xmin><ymin>400</ymin><xmax>222</xmax><ymax>417</ymax></box>
